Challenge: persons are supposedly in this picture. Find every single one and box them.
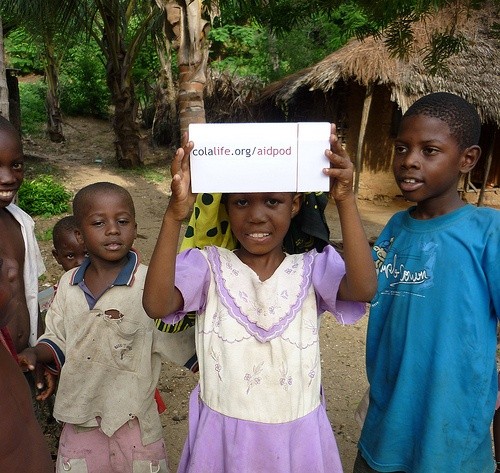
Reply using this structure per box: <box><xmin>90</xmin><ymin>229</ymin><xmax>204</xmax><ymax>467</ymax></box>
<box><xmin>0</xmin><ymin>117</ymin><xmax>378</xmax><ymax>473</ymax></box>
<box><xmin>352</xmin><ymin>92</ymin><xmax>500</xmax><ymax>473</ymax></box>
